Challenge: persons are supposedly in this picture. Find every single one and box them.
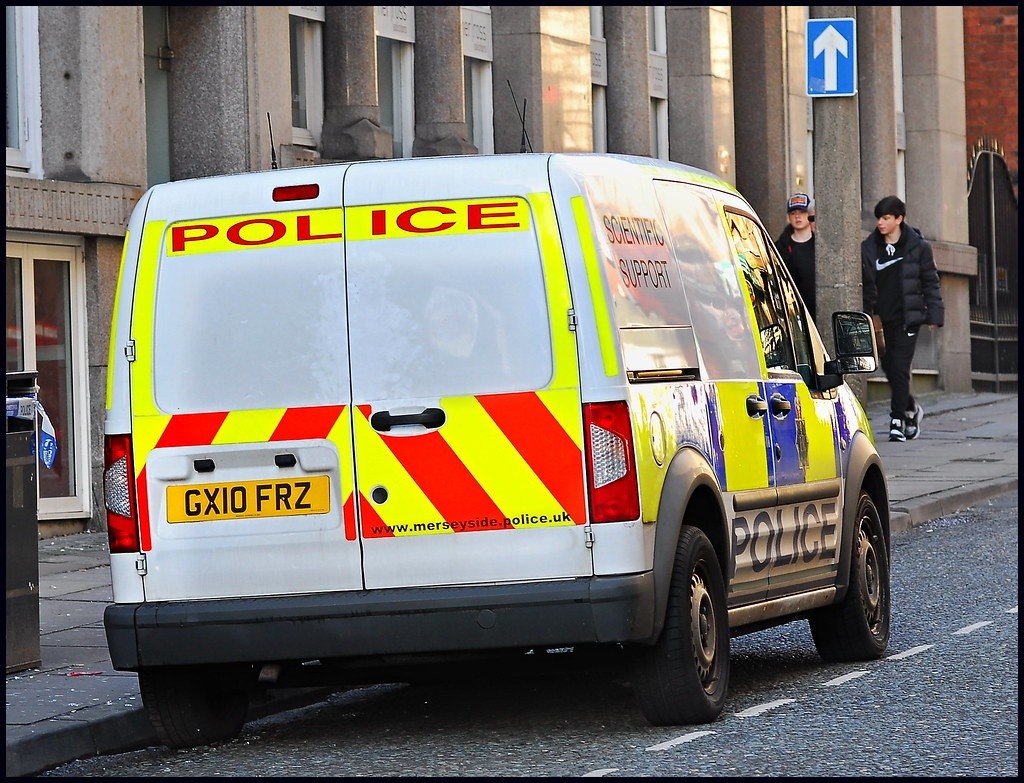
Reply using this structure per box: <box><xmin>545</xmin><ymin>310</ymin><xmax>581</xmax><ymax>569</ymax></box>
<box><xmin>774</xmin><ymin>193</ymin><xmax>817</xmax><ymax>325</ymax></box>
<box><xmin>861</xmin><ymin>196</ymin><xmax>945</xmax><ymax>442</ymax></box>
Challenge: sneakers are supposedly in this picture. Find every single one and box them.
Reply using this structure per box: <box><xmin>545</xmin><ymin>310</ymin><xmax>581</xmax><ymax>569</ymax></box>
<box><xmin>888</xmin><ymin>401</ymin><xmax>924</xmax><ymax>442</ymax></box>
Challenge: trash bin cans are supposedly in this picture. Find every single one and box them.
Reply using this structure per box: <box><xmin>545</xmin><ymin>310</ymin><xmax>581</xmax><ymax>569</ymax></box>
<box><xmin>0</xmin><ymin>368</ymin><xmax>44</xmax><ymax>673</ymax></box>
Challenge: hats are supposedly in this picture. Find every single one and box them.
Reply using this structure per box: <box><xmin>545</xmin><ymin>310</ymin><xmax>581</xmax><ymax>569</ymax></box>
<box><xmin>787</xmin><ymin>193</ymin><xmax>810</xmax><ymax>213</ymax></box>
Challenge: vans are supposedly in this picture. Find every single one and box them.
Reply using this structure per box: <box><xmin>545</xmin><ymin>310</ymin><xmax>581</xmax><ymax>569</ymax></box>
<box><xmin>103</xmin><ymin>153</ymin><xmax>894</xmax><ymax>746</ymax></box>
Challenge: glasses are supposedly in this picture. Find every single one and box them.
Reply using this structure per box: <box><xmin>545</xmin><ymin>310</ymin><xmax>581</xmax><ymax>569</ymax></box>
<box><xmin>808</xmin><ymin>215</ymin><xmax>816</xmax><ymax>222</ymax></box>
